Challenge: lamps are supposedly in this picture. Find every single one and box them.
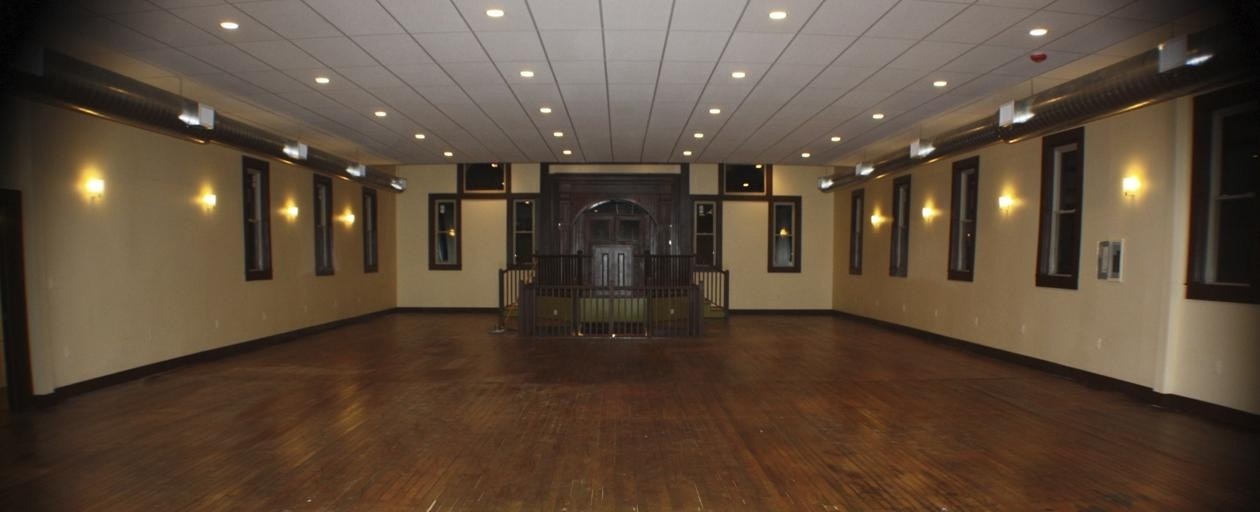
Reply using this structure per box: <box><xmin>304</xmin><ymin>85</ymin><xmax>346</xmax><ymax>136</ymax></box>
<box><xmin>991</xmin><ymin>93</ymin><xmax>1039</xmax><ymax>129</ymax></box>
<box><xmin>851</xmin><ymin>161</ymin><xmax>877</xmax><ymax>180</ymax></box>
<box><xmin>1147</xmin><ymin>34</ymin><xmax>1213</xmax><ymax>81</ymax></box>
<box><xmin>905</xmin><ymin>137</ymin><xmax>938</xmax><ymax>163</ymax></box>
<box><xmin>178</xmin><ymin>102</ymin><xmax>408</xmax><ymax>193</ymax></box>
<box><xmin>818</xmin><ymin>176</ymin><xmax>836</xmax><ymax>193</ymax></box>
